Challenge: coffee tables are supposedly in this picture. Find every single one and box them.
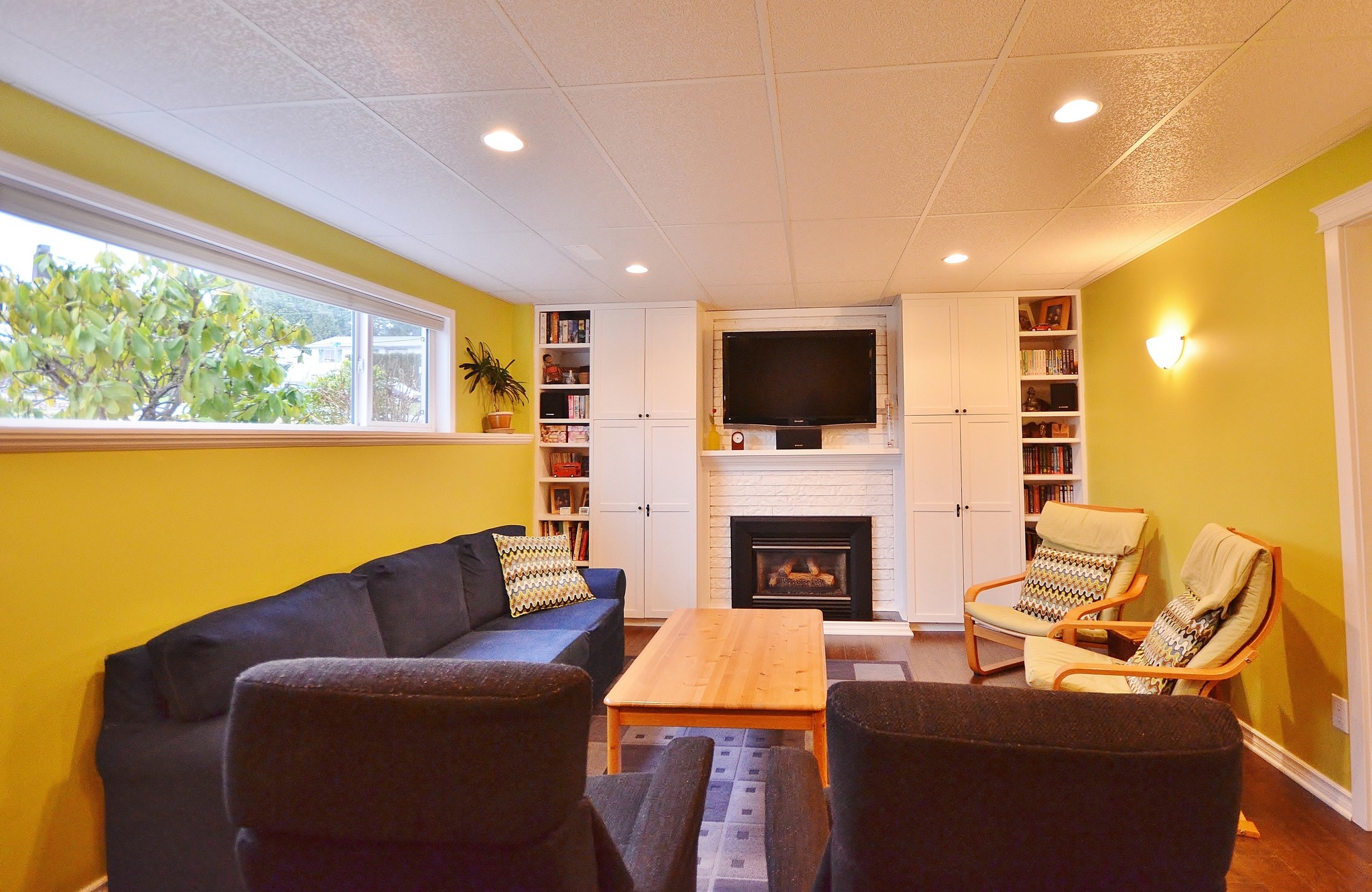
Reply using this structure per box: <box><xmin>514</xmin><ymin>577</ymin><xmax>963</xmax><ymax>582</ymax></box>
<box><xmin>603</xmin><ymin>606</ymin><xmax>830</xmax><ymax>789</ymax></box>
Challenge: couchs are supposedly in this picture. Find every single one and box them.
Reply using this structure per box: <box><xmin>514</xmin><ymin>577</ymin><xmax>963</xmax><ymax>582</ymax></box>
<box><xmin>762</xmin><ymin>679</ymin><xmax>1245</xmax><ymax>892</ymax></box>
<box><xmin>93</xmin><ymin>522</ymin><xmax>627</xmax><ymax>892</ymax></box>
<box><xmin>217</xmin><ymin>652</ymin><xmax>715</xmax><ymax>892</ymax></box>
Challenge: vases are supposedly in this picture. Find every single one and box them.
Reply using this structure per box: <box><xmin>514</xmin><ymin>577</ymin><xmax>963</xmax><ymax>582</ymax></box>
<box><xmin>707</xmin><ymin>425</ymin><xmax>720</xmax><ymax>450</ymax></box>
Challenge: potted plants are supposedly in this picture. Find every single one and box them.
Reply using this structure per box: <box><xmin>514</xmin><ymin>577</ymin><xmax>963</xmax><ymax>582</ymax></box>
<box><xmin>459</xmin><ymin>335</ymin><xmax>530</xmax><ymax>433</ymax></box>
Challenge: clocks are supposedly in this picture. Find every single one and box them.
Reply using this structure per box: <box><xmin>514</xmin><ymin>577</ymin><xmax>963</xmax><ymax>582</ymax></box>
<box><xmin>731</xmin><ymin>431</ymin><xmax>744</xmax><ymax>450</ymax></box>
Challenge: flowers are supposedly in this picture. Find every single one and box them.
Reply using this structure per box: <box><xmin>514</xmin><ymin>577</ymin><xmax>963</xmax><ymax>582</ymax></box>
<box><xmin>708</xmin><ymin>408</ymin><xmax>717</xmax><ymax>426</ymax></box>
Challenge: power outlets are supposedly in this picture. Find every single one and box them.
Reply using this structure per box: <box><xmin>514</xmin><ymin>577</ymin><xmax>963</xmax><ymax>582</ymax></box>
<box><xmin>1330</xmin><ymin>694</ymin><xmax>1350</xmax><ymax>735</ymax></box>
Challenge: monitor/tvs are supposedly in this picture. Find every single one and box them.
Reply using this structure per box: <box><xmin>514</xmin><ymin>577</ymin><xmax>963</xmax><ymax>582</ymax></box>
<box><xmin>722</xmin><ymin>329</ymin><xmax>877</xmax><ymax>430</ymax></box>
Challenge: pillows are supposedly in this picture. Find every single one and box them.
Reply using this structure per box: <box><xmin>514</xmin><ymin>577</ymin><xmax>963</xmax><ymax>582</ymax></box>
<box><xmin>1124</xmin><ymin>584</ymin><xmax>1223</xmax><ymax>696</ymax></box>
<box><xmin>492</xmin><ymin>533</ymin><xmax>596</xmax><ymax>618</ymax></box>
<box><xmin>1008</xmin><ymin>544</ymin><xmax>1119</xmax><ymax>629</ymax></box>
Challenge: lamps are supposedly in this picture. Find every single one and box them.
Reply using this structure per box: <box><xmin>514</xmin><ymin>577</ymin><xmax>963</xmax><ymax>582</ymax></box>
<box><xmin>1144</xmin><ymin>318</ymin><xmax>1191</xmax><ymax>372</ymax></box>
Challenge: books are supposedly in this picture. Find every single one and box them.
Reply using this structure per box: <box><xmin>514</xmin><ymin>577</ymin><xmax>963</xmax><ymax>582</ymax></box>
<box><xmin>1020</xmin><ymin>348</ymin><xmax>1079</xmax><ymax>560</ymax></box>
<box><xmin>538</xmin><ymin>311</ymin><xmax>592</xmax><ymax>564</ymax></box>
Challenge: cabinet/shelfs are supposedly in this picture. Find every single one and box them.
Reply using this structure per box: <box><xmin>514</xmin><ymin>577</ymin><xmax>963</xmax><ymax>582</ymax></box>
<box><xmin>532</xmin><ymin>304</ymin><xmax>711</xmax><ymax>623</ymax></box>
<box><xmin>896</xmin><ymin>291</ymin><xmax>1090</xmax><ymax>629</ymax></box>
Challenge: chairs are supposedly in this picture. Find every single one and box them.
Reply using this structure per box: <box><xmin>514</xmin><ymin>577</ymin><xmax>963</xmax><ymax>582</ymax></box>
<box><xmin>1023</xmin><ymin>523</ymin><xmax>1284</xmax><ymax>696</ymax></box>
<box><xmin>963</xmin><ymin>500</ymin><xmax>1149</xmax><ymax>678</ymax></box>
